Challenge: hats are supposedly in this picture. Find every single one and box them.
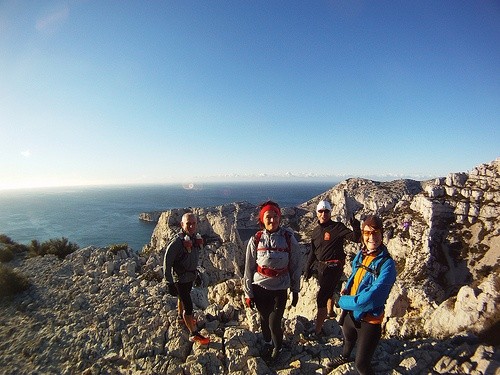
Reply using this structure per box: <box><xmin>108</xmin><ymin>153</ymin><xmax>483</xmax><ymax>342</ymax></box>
<box><xmin>317</xmin><ymin>201</ymin><xmax>331</xmax><ymax>210</ymax></box>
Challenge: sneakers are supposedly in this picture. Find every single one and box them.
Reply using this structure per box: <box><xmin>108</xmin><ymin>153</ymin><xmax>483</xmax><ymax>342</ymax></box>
<box><xmin>188</xmin><ymin>332</ymin><xmax>210</xmax><ymax>345</ymax></box>
<box><xmin>260</xmin><ymin>344</ymin><xmax>273</xmax><ymax>360</ymax></box>
<box><xmin>266</xmin><ymin>347</ymin><xmax>283</xmax><ymax>367</ymax></box>
<box><xmin>178</xmin><ymin>317</ymin><xmax>196</xmax><ymax>324</ymax></box>
<box><xmin>327</xmin><ymin>354</ymin><xmax>350</xmax><ymax>367</ymax></box>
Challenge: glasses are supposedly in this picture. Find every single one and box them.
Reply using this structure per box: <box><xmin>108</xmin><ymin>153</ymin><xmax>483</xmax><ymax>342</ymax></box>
<box><xmin>363</xmin><ymin>230</ymin><xmax>383</xmax><ymax>238</ymax></box>
<box><xmin>318</xmin><ymin>209</ymin><xmax>330</xmax><ymax>214</ymax></box>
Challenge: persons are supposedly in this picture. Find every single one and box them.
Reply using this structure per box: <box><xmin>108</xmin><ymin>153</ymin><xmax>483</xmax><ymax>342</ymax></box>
<box><xmin>327</xmin><ymin>215</ymin><xmax>398</xmax><ymax>375</ymax></box>
<box><xmin>163</xmin><ymin>212</ymin><xmax>210</xmax><ymax>344</ymax></box>
<box><xmin>243</xmin><ymin>201</ymin><xmax>301</xmax><ymax>361</ymax></box>
<box><xmin>303</xmin><ymin>201</ymin><xmax>361</xmax><ymax>340</ymax></box>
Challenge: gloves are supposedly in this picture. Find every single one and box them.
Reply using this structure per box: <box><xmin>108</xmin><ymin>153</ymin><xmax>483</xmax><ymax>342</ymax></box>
<box><xmin>169</xmin><ymin>284</ymin><xmax>179</xmax><ymax>296</ymax></box>
<box><xmin>245</xmin><ymin>298</ymin><xmax>253</xmax><ymax>308</ymax></box>
<box><xmin>195</xmin><ymin>275</ymin><xmax>202</xmax><ymax>287</ymax></box>
<box><xmin>350</xmin><ymin>213</ymin><xmax>360</xmax><ymax>230</ymax></box>
<box><xmin>334</xmin><ymin>296</ymin><xmax>340</xmax><ymax>307</ymax></box>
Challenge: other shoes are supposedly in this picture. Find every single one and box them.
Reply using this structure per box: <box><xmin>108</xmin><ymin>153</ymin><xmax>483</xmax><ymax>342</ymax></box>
<box><xmin>328</xmin><ymin>310</ymin><xmax>337</xmax><ymax>318</ymax></box>
<box><xmin>305</xmin><ymin>331</ymin><xmax>322</xmax><ymax>340</ymax></box>
